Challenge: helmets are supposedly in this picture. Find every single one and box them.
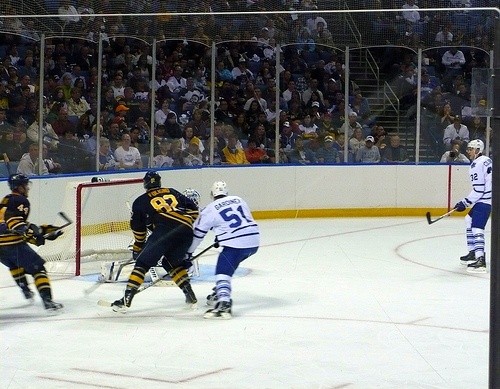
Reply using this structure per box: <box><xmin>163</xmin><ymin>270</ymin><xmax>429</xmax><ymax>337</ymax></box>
<box><xmin>8</xmin><ymin>173</ymin><xmax>29</xmax><ymax>193</ymax></box>
<box><xmin>467</xmin><ymin>139</ymin><xmax>484</xmax><ymax>158</ymax></box>
<box><xmin>213</xmin><ymin>181</ymin><xmax>229</xmax><ymax>198</ymax></box>
<box><xmin>144</xmin><ymin>171</ymin><xmax>162</xmax><ymax>190</ymax></box>
<box><xmin>183</xmin><ymin>189</ymin><xmax>199</xmax><ymax>207</ymax></box>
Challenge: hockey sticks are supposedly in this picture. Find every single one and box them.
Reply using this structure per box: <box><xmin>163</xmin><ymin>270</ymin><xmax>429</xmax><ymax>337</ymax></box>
<box><xmin>425</xmin><ymin>208</ymin><xmax>457</xmax><ymax>225</ymax></box>
<box><xmin>149</xmin><ymin>267</ymin><xmax>179</xmax><ymax>286</ymax></box>
<box><xmin>97</xmin><ymin>241</ymin><xmax>218</xmax><ymax>312</ymax></box>
<box><xmin>109</xmin><ymin>257</ymin><xmax>133</xmax><ymax>282</ymax></box>
<box><xmin>124</xmin><ymin>199</ymin><xmax>152</xmax><ymax>235</ymax></box>
<box><xmin>115</xmin><ymin>259</ymin><xmax>136</xmax><ymax>282</ymax></box>
<box><xmin>42</xmin><ymin>212</ymin><xmax>73</xmax><ymax>239</ymax></box>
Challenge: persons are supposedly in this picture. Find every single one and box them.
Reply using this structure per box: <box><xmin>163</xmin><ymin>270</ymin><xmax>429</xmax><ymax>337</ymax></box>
<box><xmin>0</xmin><ymin>0</ymin><xmax>495</xmax><ymax>163</ymax></box>
<box><xmin>455</xmin><ymin>139</ymin><xmax>492</xmax><ymax>273</ymax></box>
<box><xmin>111</xmin><ymin>171</ymin><xmax>260</xmax><ymax>319</ymax></box>
<box><xmin>0</xmin><ymin>174</ymin><xmax>65</xmax><ymax>314</ymax></box>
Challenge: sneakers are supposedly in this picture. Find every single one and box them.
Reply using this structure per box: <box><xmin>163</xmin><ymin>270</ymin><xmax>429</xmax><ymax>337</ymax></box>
<box><xmin>207</xmin><ymin>293</ymin><xmax>218</xmax><ymax>306</ymax></box>
<box><xmin>183</xmin><ymin>284</ymin><xmax>199</xmax><ymax>309</ymax></box>
<box><xmin>459</xmin><ymin>249</ymin><xmax>487</xmax><ymax>273</ymax></box>
<box><xmin>24</xmin><ymin>291</ymin><xmax>36</xmax><ymax>305</ymax></box>
<box><xmin>204</xmin><ymin>300</ymin><xmax>232</xmax><ymax>319</ymax></box>
<box><xmin>111</xmin><ymin>289</ymin><xmax>135</xmax><ymax>314</ymax></box>
<box><xmin>44</xmin><ymin>298</ymin><xmax>64</xmax><ymax>315</ymax></box>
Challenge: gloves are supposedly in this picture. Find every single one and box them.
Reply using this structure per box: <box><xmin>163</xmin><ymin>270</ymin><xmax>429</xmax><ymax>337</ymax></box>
<box><xmin>36</xmin><ymin>225</ymin><xmax>65</xmax><ymax>247</ymax></box>
<box><xmin>454</xmin><ymin>202</ymin><xmax>465</xmax><ymax>212</ymax></box>
<box><xmin>132</xmin><ymin>251</ymin><xmax>142</xmax><ymax>260</ymax></box>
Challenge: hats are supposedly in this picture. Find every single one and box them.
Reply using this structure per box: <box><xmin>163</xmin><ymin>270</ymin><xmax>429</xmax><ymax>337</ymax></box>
<box><xmin>116</xmin><ymin>105</ymin><xmax>129</xmax><ymax>112</ymax></box>
<box><xmin>324</xmin><ymin>136</ymin><xmax>334</xmax><ymax>143</ymax></box>
<box><xmin>329</xmin><ymin>78</ymin><xmax>336</xmax><ymax>84</ymax></box>
<box><xmin>365</xmin><ymin>136</ymin><xmax>375</xmax><ymax>143</ymax></box>
<box><xmin>282</xmin><ymin>121</ymin><xmax>291</xmax><ymax>128</ymax></box>
<box><xmin>239</xmin><ymin>58</ymin><xmax>246</xmax><ymax>63</ymax></box>
<box><xmin>349</xmin><ymin>112</ymin><xmax>357</xmax><ymax>117</ymax></box>
<box><xmin>261</xmin><ymin>27</ymin><xmax>269</xmax><ymax>32</ymax></box>
<box><xmin>479</xmin><ymin>100</ymin><xmax>486</xmax><ymax>106</ymax></box>
<box><xmin>454</xmin><ymin>115</ymin><xmax>462</xmax><ymax>121</ymax></box>
<box><xmin>312</xmin><ymin>102</ymin><xmax>319</xmax><ymax>107</ymax></box>
